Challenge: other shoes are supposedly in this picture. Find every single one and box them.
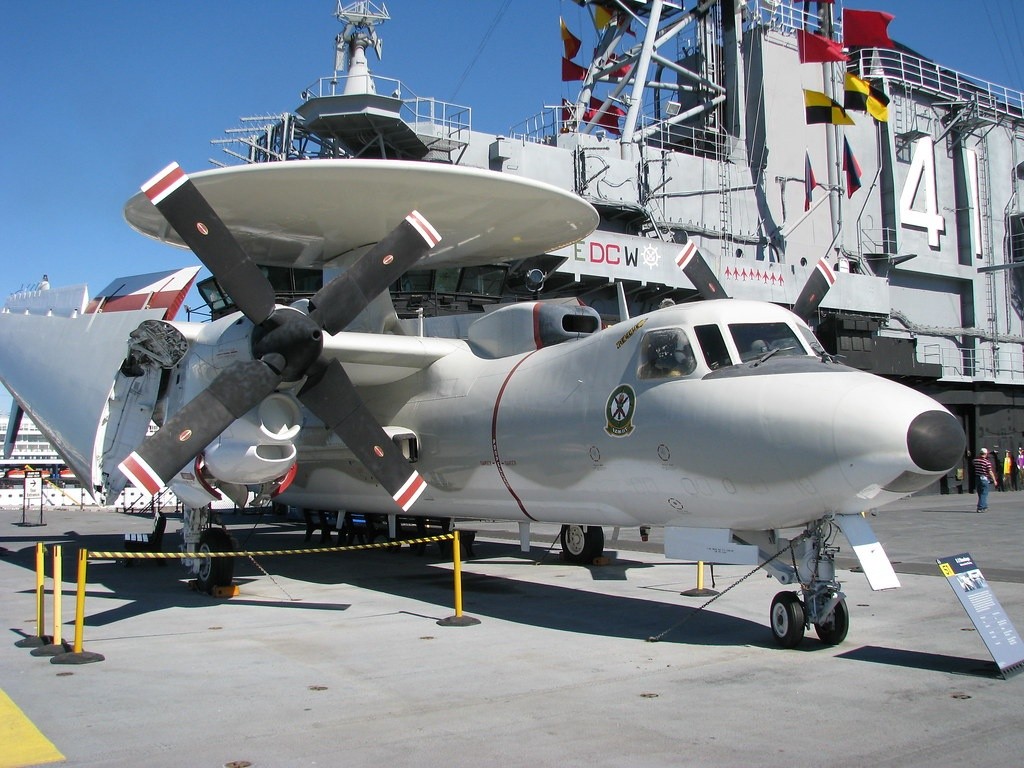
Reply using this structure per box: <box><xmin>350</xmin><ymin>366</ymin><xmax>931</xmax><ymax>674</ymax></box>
<box><xmin>977</xmin><ymin>509</ymin><xmax>985</xmax><ymax>512</ymax></box>
<box><xmin>984</xmin><ymin>507</ymin><xmax>990</xmax><ymax>511</ymax></box>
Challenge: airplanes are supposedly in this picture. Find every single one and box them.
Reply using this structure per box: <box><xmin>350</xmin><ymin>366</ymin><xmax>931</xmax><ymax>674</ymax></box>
<box><xmin>1</xmin><ymin>160</ymin><xmax>967</xmax><ymax>648</ymax></box>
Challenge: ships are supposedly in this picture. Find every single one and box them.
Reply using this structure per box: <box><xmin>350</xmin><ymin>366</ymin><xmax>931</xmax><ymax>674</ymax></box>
<box><xmin>184</xmin><ymin>0</ymin><xmax>1024</xmax><ymax>393</ymax></box>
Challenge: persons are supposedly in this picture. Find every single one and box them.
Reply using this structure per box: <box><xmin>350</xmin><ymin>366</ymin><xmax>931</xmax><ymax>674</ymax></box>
<box><xmin>972</xmin><ymin>446</ymin><xmax>1024</xmax><ymax>514</ymax></box>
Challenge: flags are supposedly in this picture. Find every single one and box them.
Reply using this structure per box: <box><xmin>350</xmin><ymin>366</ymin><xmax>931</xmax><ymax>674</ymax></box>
<box><xmin>796</xmin><ymin>29</ymin><xmax>850</xmax><ymax>65</ymax></box>
<box><xmin>842</xmin><ymin>134</ymin><xmax>862</xmax><ymax>199</ymax></box>
<box><xmin>802</xmin><ymin>88</ymin><xmax>856</xmax><ymax>125</ymax></box>
<box><xmin>559</xmin><ymin>5</ymin><xmax>634</xmax><ymax>136</ymax></box>
<box><xmin>804</xmin><ymin>151</ymin><xmax>817</xmax><ymax>212</ymax></box>
<box><xmin>841</xmin><ymin>8</ymin><xmax>896</xmax><ymax>50</ymax></box>
<box><xmin>843</xmin><ymin>72</ymin><xmax>891</xmax><ymax>122</ymax></box>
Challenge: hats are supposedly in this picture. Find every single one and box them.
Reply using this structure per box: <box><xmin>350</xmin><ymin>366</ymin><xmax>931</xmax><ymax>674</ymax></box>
<box><xmin>980</xmin><ymin>448</ymin><xmax>988</xmax><ymax>454</ymax></box>
